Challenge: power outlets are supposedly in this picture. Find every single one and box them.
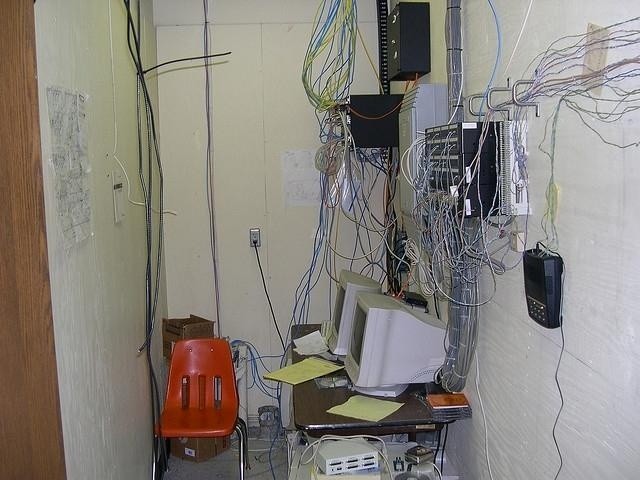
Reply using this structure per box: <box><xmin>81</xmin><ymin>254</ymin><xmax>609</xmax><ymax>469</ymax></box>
<box><xmin>249</xmin><ymin>227</ymin><xmax>261</xmax><ymax>248</ymax></box>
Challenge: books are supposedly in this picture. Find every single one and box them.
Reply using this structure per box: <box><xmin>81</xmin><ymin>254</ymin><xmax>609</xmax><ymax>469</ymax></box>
<box><xmin>427</xmin><ymin>393</ymin><xmax>469</xmax><ymax>409</ymax></box>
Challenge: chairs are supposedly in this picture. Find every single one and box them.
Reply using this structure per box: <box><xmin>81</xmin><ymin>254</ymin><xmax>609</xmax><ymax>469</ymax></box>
<box><xmin>152</xmin><ymin>338</ymin><xmax>250</xmax><ymax>480</ymax></box>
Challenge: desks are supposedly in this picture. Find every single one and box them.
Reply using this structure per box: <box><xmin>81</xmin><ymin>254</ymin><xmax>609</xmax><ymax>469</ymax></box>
<box><xmin>291</xmin><ymin>323</ymin><xmax>448</xmax><ymax>475</ymax></box>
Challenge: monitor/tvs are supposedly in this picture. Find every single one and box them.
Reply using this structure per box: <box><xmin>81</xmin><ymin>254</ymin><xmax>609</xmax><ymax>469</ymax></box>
<box><xmin>327</xmin><ymin>269</ymin><xmax>426</xmax><ymax>361</ymax></box>
<box><xmin>344</xmin><ymin>290</ymin><xmax>449</xmax><ymax>398</ymax></box>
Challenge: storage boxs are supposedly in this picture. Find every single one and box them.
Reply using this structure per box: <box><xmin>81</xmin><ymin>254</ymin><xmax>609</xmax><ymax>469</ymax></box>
<box><xmin>160</xmin><ymin>312</ymin><xmax>215</xmax><ymax>359</ymax></box>
<box><xmin>170</xmin><ymin>435</ymin><xmax>230</xmax><ymax>462</ymax></box>
